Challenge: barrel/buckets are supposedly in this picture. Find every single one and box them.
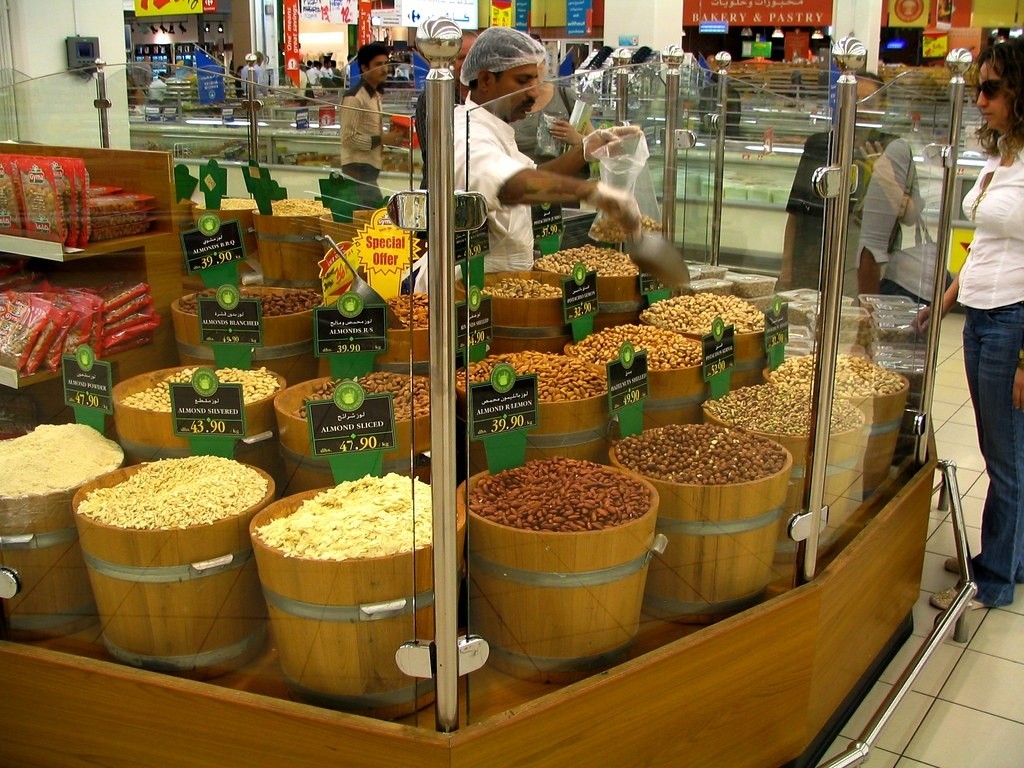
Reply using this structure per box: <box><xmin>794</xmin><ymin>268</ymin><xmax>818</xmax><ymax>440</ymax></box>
<box><xmin>0</xmin><ymin>198</ymin><xmax>910</xmax><ymax>721</ymax></box>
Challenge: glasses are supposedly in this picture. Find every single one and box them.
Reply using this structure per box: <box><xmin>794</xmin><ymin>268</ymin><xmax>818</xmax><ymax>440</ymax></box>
<box><xmin>970</xmin><ymin>170</ymin><xmax>994</xmax><ymax>211</ymax></box>
<box><xmin>862</xmin><ymin>96</ymin><xmax>876</xmax><ymax>108</ymax></box>
<box><xmin>976</xmin><ymin>79</ymin><xmax>1007</xmax><ymax>100</ymax></box>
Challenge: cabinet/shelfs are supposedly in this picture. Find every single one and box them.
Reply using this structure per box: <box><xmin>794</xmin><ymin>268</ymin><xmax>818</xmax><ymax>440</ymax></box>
<box><xmin>0</xmin><ymin>140</ymin><xmax>184</xmax><ymax>391</ymax></box>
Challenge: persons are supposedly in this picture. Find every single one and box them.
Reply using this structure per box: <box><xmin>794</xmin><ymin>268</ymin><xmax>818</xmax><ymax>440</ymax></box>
<box><xmin>343</xmin><ymin>55</ymin><xmax>354</xmax><ymax>88</ymax></box>
<box><xmin>454</xmin><ymin>27</ymin><xmax>642</xmax><ymax>270</ymax></box>
<box><xmin>235</xmin><ymin>52</ymin><xmax>268</xmax><ymax>98</ymax></box>
<box><xmin>858</xmin><ymin>77</ymin><xmax>953</xmax><ymax>497</ymax></box>
<box><xmin>509</xmin><ymin>34</ymin><xmax>596</xmax><ymax>210</ymax></box>
<box><xmin>415</xmin><ymin>29</ymin><xmax>477</xmax><ymax>189</ymax></box>
<box><xmin>301</xmin><ymin>58</ymin><xmax>340</xmax><ymax>88</ymax></box>
<box><xmin>341</xmin><ymin>41</ymin><xmax>404</xmax><ymax>199</ymax></box>
<box><xmin>912</xmin><ymin>43</ymin><xmax>1024</xmax><ymax>612</ymax></box>
<box><xmin>149</xmin><ymin>71</ymin><xmax>167</xmax><ymax>104</ymax></box>
<box><xmin>780</xmin><ymin>73</ymin><xmax>925</xmax><ymax>294</ymax></box>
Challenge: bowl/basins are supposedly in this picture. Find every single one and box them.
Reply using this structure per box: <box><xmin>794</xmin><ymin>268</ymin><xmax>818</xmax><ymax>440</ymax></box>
<box><xmin>628</xmin><ymin>232</ymin><xmax>691</xmax><ymax>296</ymax></box>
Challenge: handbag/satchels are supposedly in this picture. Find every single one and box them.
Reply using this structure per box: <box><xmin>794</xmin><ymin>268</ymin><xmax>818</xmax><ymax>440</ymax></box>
<box><xmin>853</xmin><ymin>137</ymin><xmax>916</xmax><ymax>253</ymax></box>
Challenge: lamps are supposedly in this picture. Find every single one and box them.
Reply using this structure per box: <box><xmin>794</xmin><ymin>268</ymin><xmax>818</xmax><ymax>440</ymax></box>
<box><xmin>204</xmin><ymin>23</ymin><xmax>211</xmax><ymax>32</ymax></box>
<box><xmin>741</xmin><ymin>27</ymin><xmax>753</xmax><ymax>36</ymax></box>
<box><xmin>811</xmin><ymin>30</ymin><xmax>823</xmax><ymax>39</ymax></box>
<box><xmin>160</xmin><ymin>23</ymin><xmax>168</xmax><ymax>34</ymax></box>
<box><xmin>179</xmin><ymin>23</ymin><xmax>187</xmax><ymax>33</ymax></box>
<box><xmin>772</xmin><ymin>26</ymin><xmax>784</xmax><ymax>38</ymax></box>
<box><xmin>149</xmin><ymin>24</ymin><xmax>158</xmax><ymax>34</ymax></box>
<box><xmin>168</xmin><ymin>24</ymin><xmax>176</xmax><ymax>34</ymax></box>
<box><xmin>217</xmin><ymin>22</ymin><xmax>224</xmax><ymax>33</ymax></box>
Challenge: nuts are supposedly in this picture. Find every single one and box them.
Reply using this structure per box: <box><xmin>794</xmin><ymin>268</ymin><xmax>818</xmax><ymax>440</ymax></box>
<box><xmin>114</xmin><ymin>208</ymin><xmax>905</xmax><ymax>534</ymax></box>
<box><xmin>0</xmin><ymin>171</ymin><xmax>158</xmax><ymax>377</ymax></box>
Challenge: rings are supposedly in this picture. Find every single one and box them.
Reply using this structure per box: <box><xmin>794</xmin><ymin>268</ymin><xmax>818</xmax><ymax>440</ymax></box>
<box><xmin>563</xmin><ymin>132</ymin><xmax>568</xmax><ymax>137</ymax></box>
<box><xmin>396</xmin><ymin>138</ymin><xmax>397</xmax><ymax>140</ymax></box>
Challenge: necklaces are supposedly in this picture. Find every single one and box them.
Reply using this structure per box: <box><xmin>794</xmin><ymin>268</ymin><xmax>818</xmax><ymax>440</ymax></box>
<box><xmin>970</xmin><ymin>192</ymin><xmax>987</xmax><ymax>218</ymax></box>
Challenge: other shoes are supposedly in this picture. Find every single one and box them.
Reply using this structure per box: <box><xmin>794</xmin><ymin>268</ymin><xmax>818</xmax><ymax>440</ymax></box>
<box><xmin>945</xmin><ymin>555</ymin><xmax>960</xmax><ymax>573</ymax></box>
<box><xmin>929</xmin><ymin>586</ymin><xmax>998</xmax><ymax>610</ymax></box>
